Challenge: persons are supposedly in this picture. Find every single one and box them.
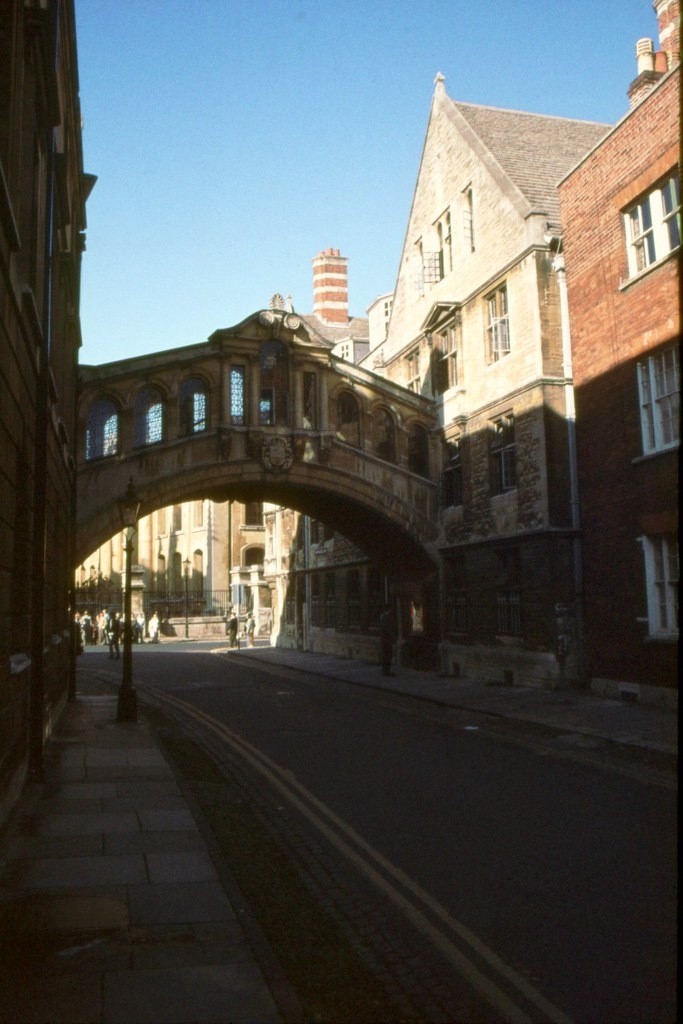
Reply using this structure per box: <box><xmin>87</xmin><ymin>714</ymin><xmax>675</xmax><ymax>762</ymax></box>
<box><xmin>226</xmin><ymin>612</ymin><xmax>254</xmax><ymax>650</ymax></box>
<box><xmin>75</xmin><ymin>609</ymin><xmax>161</xmax><ymax>660</ymax></box>
<box><xmin>380</xmin><ymin>602</ymin><xmax>396</xmax><ymax>675</ymax></box>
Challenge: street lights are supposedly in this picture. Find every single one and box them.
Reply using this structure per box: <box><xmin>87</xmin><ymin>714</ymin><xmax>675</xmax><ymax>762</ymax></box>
<box><xmin>112</xmin><ymin>474</ymin><xmax>146</xmax><ymax>723</ymax></box>
